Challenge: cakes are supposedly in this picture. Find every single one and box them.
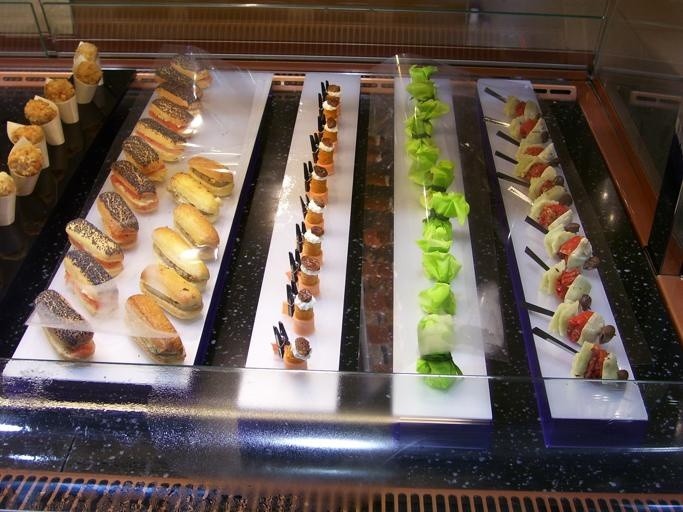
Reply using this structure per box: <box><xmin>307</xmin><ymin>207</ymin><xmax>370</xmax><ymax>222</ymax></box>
<box><xmin>310</xmin><ymin>134</ymin><xmax>337</xmax><ymax>175</ymax></box>
<box><xmin>295</xmin><ymin>224</ymin><xmax>324</xmax><ymax>261</ymax></box>
<box><xmin>288</xmin><ymin>252</ymin><xmax>320</xmax><ymax>298</ymax></box>
<box><xmin>321</xmin><ymin>81</ymin><xmax>341</xmax><ymax>111</ymax></box>
<box><xmin>317</xmin><ymin>113</ymin><xmax>338</xmax><ymax>145</ymax></box>
<box><xmin>317</xmin><ymin>95</ymin><xmax>339</xmax><ymax>125</ymax></box>
<box><xmin>272</xmin><ymin>324</ymin><xmax>310</xmax><ymax>372</ymax></box>
<box><xmin>300</xmin><ymin>196</ymin><xmax>325</xmax><ymax>233</ymax></box>
<box><xmin>304</xmin><ymin>164</ymin><xmax>328</xmax><ymax>206</ymax></box>
<box><xmin>282</xmin><ymin>285</ymin><xmax>314</xmax><ymax>334</ymax></box>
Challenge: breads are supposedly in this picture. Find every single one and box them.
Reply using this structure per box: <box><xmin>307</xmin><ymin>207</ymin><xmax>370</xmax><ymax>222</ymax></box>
<box><xmin>174</xmin><ymin>204</ymin><xmax>219</xmax><ymax>262</ymax></box>
<box><xmin>189</xmin><ymin>157</ymin><xmax>234</xmax><ymax>197</ymax></box>
<box><xmin>156</xmin><ymin>82</ymin><xmax>202</xmax><ymax>116</ymax></box>
<box><xmin>171</xmin><ymin>56</ymin><xmax>212</xmax><ymax>89</ymax></box>
<box><xmin>149</xmin><ymin>99</ymin><xmax>196</xmax><ymax>139</ymax></box>
<box><xmin>66</xmin><ymin>219</ymin><xmax>124</xmax><ymax>280</ymax></box>
<box><xmin>136</xmin><ymin>119</ymin><xmax>185</xmax><ymax>163</ymax></box>
<box><xmin>155</xmin><ymin>65</ymin><xmax>202</xmax><ymax>102</ymax></box>
<box><xmin>37</xmin><ymin>291</ymin><xmax>96</xmax><ymax>364</ymax></box>
<box><xmin>112</xmin><ymin>161</ymin><xmax>158</xmax><ymax>215</ymax></box>
<box><xmin>127</xmin><ymin>295</ymin><xmax>189</xmax><ymax>366</ymax></box>
<box><xmin>123</xmin><ymin>137</ymin><xmax>165</xmax><ymax>184</ymax></box>
<box><xmin>170</xmin><ymin>174</ymin><xmax>221</xmax><ymax>223</ymax></box>
<box><xmin>140</xmin><ymin>264</ymin><xmax>203</xmax><ymax>321</ymax></box>
<box><xmin>65</xmin><ymin>251</ymin><xmax>118</xmax><ymax>319</ymax></box>
<box><xmin>97</xmin><ymin>191</ymin><xmax>139</xmax><ymax>248</ymax></box>
<box><xmin>153</xmin><ymin>227</ymin><xmax>209</xmax><ymax>291</ymax></box>
